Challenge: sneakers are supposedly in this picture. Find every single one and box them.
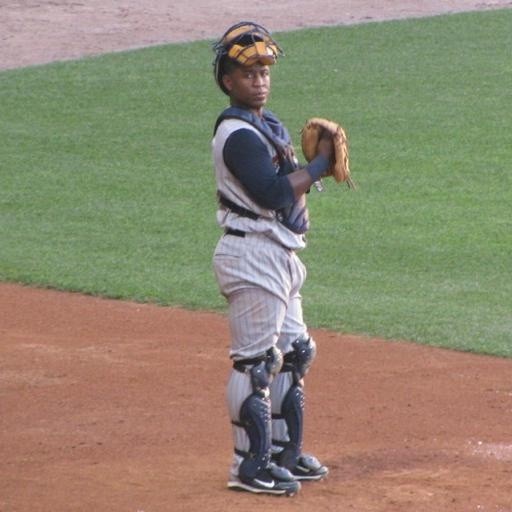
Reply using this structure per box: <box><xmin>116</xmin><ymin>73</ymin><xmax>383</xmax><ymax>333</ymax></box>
<box><xmin>292</xmin><ymin>459</ymin><xmax>332</xmax><ymax>480</ymax></box>
<box><xmin>226</xmin><ymin>472</ymin><xmax>299</xmax><ymax>494</ymax></box>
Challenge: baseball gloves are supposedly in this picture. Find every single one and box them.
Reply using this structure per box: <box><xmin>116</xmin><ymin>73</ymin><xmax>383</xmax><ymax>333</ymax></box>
<box><xmin>301</xmin><ymin>119</ymin><xmax>351</xmax><ymax>182</ymax></box>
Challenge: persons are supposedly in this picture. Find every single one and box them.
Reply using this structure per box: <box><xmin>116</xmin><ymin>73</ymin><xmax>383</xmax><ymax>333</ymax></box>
<box><xmin>212</xmin><ymin>56</ymin><xmax>333</xmax><ymax>495</ymax></box>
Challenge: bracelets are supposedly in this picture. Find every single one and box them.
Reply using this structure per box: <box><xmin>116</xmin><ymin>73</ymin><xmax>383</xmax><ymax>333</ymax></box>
<box><xmin>305</xmin><ymin>154</ymin><xmax>330</xmax><ymax>183</ymax></box>
